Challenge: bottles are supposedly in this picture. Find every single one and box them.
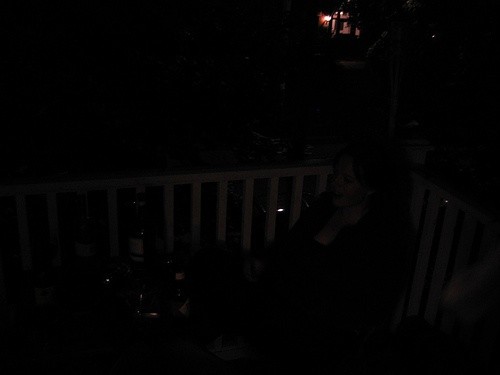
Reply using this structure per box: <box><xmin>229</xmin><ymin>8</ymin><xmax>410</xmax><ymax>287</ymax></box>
<box><xmin>70</xmin><ymin>191</ymin><xmax>97</xmax><ymax>283</ymax></box>
<box><xmin>139</xmin><ymin>268</ymin><xmax>191</xmax><ymax>321</ymax></box>
<box><xmin>126</xmin><ymin>200</ymin><xmax>155</xmax><ymax>295</ymax></box>
<box><xmin>31</xmin><ymin>271</ymin><xmax>56</xmax><ymax>309</ymax></box>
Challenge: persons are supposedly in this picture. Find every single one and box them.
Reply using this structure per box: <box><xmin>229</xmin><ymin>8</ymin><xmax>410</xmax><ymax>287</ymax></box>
<box><xmin>183</xmin><ymin>144</ymin><xmax>410</xmax><ymax>358</ymax></box>
